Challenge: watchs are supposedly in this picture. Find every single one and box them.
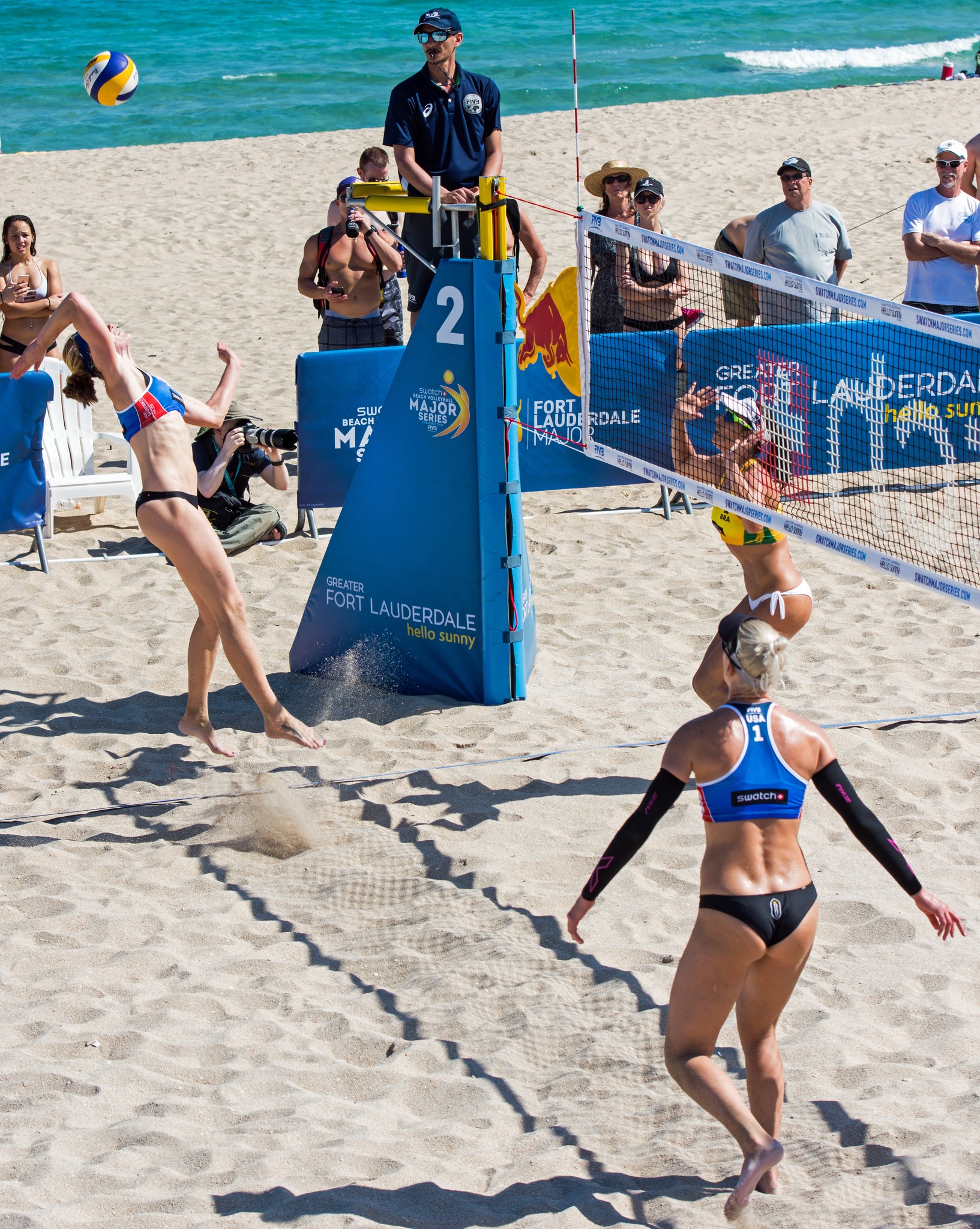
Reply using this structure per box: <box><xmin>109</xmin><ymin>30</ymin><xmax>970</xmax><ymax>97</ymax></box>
<box><xmin>271</xmin><ymin>455</ymin><xmax>284</xmax><ymax>466</ymax></box>
<box><xmin>365</xmin><ymin>225</ymin><xmax>376</xmax><ymax>238</ymax></box>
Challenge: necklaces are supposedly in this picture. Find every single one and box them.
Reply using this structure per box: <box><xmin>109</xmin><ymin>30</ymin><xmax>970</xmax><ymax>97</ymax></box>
<box><xmin>337</xmin><ymin>223</ymin><xmax>358</xmax><ymax>243</ymax></box>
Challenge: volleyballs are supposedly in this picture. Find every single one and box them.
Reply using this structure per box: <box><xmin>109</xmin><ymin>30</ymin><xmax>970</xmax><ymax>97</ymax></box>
<box><xmin>84</xmin><ymin>50</ymin><xmax>139</xmax><ymax>106</ymax></box>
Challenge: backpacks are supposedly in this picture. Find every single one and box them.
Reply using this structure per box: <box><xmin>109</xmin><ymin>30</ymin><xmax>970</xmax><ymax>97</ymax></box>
<box><xmin>312</xmin><ymin>226</ymin><xmax>386</xmax><ymax>318</ymax></box>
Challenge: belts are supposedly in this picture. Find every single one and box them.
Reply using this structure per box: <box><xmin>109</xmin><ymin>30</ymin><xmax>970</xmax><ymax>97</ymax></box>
<box><xmin>719</xmin><ymin>230</ymin><xmax>738</xmax><ymax>254</ymax></box>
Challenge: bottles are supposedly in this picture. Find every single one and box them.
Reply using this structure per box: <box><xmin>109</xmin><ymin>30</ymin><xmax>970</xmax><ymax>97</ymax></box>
<box><xmin>397</xmin><ymin>241</ymin><xmax>406</xmax><ymax>278</ymax></box>
<box><xmin>346</xmin><ymin>207</ymin><xmax>359</xmax><ymax>238</ymax></box>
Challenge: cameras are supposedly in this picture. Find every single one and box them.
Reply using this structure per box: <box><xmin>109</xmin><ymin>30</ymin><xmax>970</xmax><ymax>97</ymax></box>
<box><xmin>222</xmin><ymin>421</ymin><xmax>298</xmax><ymax>454</ymax></box>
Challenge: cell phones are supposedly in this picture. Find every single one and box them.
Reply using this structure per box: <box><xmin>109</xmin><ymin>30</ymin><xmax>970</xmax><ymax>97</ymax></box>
<box><xmin>331</xmin><ymin>287</ymin><xmax>345</xmax><ymax>297</ymax></box>
<box><xmin>17</xmin><ymin>274</ymin><xmax>29</xmax><ymax>296</ymax></box>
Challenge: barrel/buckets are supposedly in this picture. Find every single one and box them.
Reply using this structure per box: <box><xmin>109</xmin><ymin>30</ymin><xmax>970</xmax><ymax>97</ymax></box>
<box><xmin>941</xmin><ymin>57</ymin><xmax>954</xmax><ymax>80</ymax></box>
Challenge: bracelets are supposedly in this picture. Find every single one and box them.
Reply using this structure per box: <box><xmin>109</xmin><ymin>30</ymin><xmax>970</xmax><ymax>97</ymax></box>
<box><xmin>665</xmin><ymin>288</ymin><xmax>666</xmax><ymax>298</ymax></box>
<box><xmin>46</xmin><ymin>297</ymin><xmax>50</xmax><ymax>308</ymax></box>
<box><xmin>667</xmin><ymin>288</ymin><xmax>668</xmax><ymax>290</ymax></box>
<box><xmin>1</xmin><ymin>293</ymin><xmax>4</xmax><ymax>304</ymax></box>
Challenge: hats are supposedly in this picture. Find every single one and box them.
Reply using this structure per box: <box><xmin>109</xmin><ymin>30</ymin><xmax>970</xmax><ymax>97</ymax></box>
<box><xmin>413</xmin><ymin>7</ymin><xmax>461</xmax><ymax>35</ymax></box>
<box><xmin>719</xmin><ymin>614</ymin><xmax>773</xmax><ymax>688</ymax></box>
<box><xmin>75</xmin><ymin>331</ymin><xmax>92</xmax><ymax>371</ymax></box>
<box><xmin>777</xmin><ymin>157</ymin><xmax>811</xmax><ymax>177</ymax></box>
<box><xmin>194</xmin><ymin>400</ymin><xmax>262</xmax><ymax>441</ymax></box>
<box><xmin>584</xmin><ymin>159</ymin><xmax>649</xmax><ymax>197</ymax></box>
<box><xmin>337</xmin><ymin>176</ymin><xmax>364</xmax><ymax>192</ymax></box>
<box><xmin>935</xmin><ymin>140</ymin><xmax>968</xmax><ymax>161</ymax></box>
<box><xmin>721</xmin><ymin>391</ymin><xmax>770</xmax><ymax>447</ymax></box>
<box><xmin>634</xmin><ymin>177</ymin><xmax>664</xmax><ymax>198</ymax></box>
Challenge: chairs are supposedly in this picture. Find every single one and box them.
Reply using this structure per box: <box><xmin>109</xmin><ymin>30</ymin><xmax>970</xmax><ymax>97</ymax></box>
<box><xmin>0</xmin><ymin>356</ymin><xmax>144</xmax><ymax>574</ymax></box>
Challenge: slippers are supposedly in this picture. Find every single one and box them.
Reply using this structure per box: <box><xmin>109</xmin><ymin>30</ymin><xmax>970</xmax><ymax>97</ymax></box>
<box><xmin>263</xmin><ymin>521</ymin><xmax>287</xmax><ymax>543</ymax></box>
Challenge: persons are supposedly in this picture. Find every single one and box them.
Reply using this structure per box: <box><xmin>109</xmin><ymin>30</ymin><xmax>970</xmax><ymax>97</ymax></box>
<box><xmin>191</xmin><ymin>399</ymin><xmax>289</xmax><ymax>555</ymax></box>
<box><xmin>10</xmin><ymin>290</ymin><xmax>327</xmax><ymax>757</ymax></box>
<box><xmin>901</xmin><ymin>133</ymin><xmax>980</xmax><ymax>314</ymax></box>
<box><xmin>505</xmin><ymin>199</ymin><xmax>548</xmax><ymax>309</ymax></box>
<box><xmin>0</xmin><ymin>281</ymin><xmax>30</xmax><ymax>305</ymax></box>
<box><xmin>0</xmin><ymin>215</ymin><xmax>63</xmax><ymax>374</ymax></box>
<box><xmin>671</xmin><ymin>382</ymin><xmax>814</xmax><ymax>711</ymax></box>
<box><xmin>714</xmin><ymin>214</ymin><xmax>761</xmax><ymax>328</ymax></box>
<box><xmin>381</xmin><ymin>6</ymin><xmax>503</xmax><ymax>329</ymax></box>
<box><xmin>584</xmin><ymin>160</ymin><xmax>703</xmax><ymax>370</ymax></box>
<box><xmin>568</xmin><ymin>612</ymin><xmax>967</xmax><ymax>1222</ymax></box>
<box><xmin>298</xmin><ymin>146</ymin><xmax>404</xmax><ymax>352</ymax></box>
<box><xmin>743</xmin><ymin>157</ymin><xmax>852</xmax><ymax>326</ymax></box>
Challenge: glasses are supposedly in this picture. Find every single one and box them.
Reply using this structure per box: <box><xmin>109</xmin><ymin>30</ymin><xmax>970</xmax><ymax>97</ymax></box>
<box><xmin>338</xmin><ymin>195</ymin><xmax>346</xmax><ymax>202</ymax></box>
<box><xmin>417</xmin><ymin>31</ymin><xmax>459</xmax><ymax>43</ymax></box>
<box><xmin>604</xmin><ymin>175</ymin><xmax>629</xmax><ymax>183</ymax></box>
<box><xmin>936</xmin><ymin>160</ymin><xmax>964</xmax><ymax>168</ymax></box>
<box><xmin>363</xmin><ymin>173</ymin><xmax>390</xmax><ymax>183</ymax></box>
<box><xmin>636</xmin><ymin>194</ymin><xmax>659</xmax><ymax>204</ymax></box>
<box><xmin>722</xmin><ymin>408</ymin><xmax>752</xmax><ymax>431</ymax></box>
<box><xmin>721</xmin><ymin>641</ymin><xmax>741</xmax><ymax>671</ymax></box>
<box><xmin>780</xmin><ymin>173</ymin><xmax>809</xmax><ymax>181</ymax></box>
